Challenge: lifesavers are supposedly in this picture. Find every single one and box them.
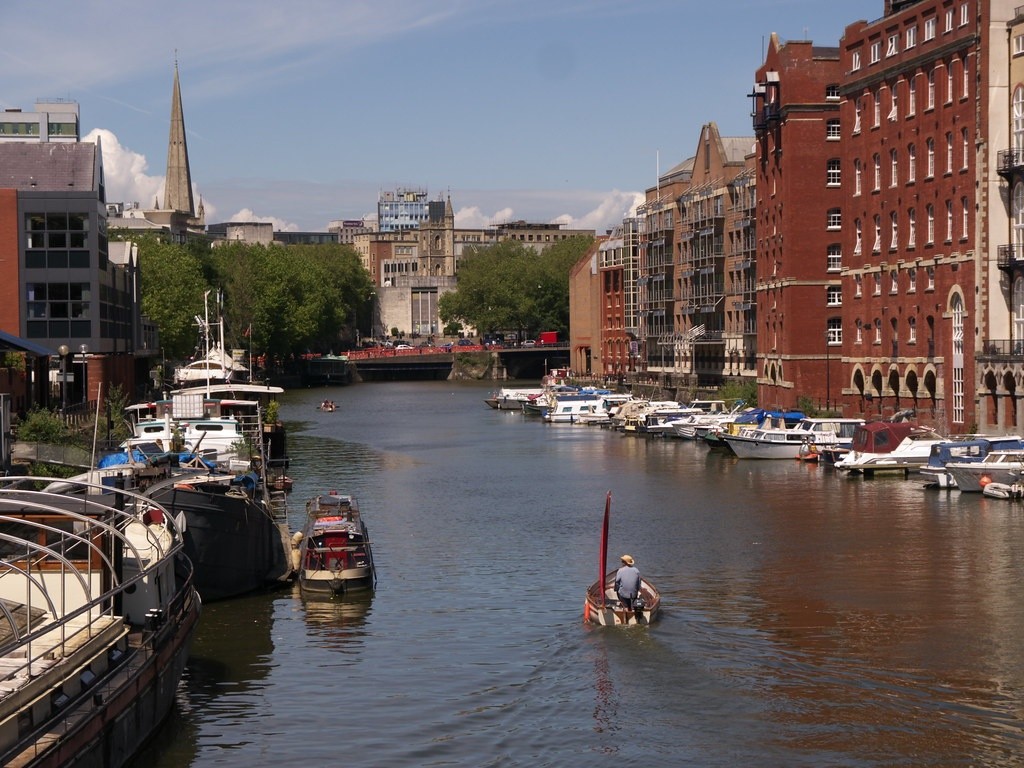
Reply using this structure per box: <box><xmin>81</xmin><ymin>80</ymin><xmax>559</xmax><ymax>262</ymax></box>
<box><xmin>317</xmin><ymin>515</ymin><xmax>342</xmax><ymax>523</ymax></box>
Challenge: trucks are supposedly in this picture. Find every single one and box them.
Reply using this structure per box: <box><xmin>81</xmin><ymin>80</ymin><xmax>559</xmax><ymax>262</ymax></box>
<box><xmin>534</xmin><ymin>331</ymin><xmax>558</xmax><ymax>348</ymax></box>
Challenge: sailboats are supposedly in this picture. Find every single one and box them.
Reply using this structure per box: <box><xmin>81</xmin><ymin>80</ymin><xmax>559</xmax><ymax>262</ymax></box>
<box><xmin>583</xmin><ymin>490</ymin><xmax>661</xmax><ymax>628</ymax></box>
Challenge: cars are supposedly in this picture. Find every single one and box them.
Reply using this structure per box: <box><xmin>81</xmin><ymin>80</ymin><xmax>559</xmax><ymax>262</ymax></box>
<box><xmin>523</xmin><ymin>339</ymin><xmax>536</xmax><ymax>348</ymax></box>
<box><xmin>419</xmin><ymin>340</ymin><xmax>436</xmax><ymax>347</ymax></box>
<box><xmin>380</xmin><ymin>339</ymin><xmax>416</xmax><ymax>349</ymax></box>
<box><xmin>458</xmin><ymin>340</ymin><xmax>475</xmax><ymax>346</ymax></box>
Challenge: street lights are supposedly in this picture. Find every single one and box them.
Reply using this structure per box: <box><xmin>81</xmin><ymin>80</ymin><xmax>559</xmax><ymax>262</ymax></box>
<box><xmin>56</xmin><ymin>344</ymin><xmax>69</xmax><ymax>423</ymax></box>
<box><xmin>78</xmin><ymin>343</ymin><xmax>89</xmax><ymax>416</ymax></box>
<box><xmin>371</xmin><ymin>279</ymin><xmax>376</xmax><ymax>349</ymax></box>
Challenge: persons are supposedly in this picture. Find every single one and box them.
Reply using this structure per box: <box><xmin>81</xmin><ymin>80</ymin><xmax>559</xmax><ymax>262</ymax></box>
<box><xmin>649</xmin><ymin>373</ymin><xmax>658</xmax><ymax>384</ymax></box>
<box><xmin>663</xmin><ymin>373</ymin><xmax>672</xmax><ymax>387</ymax></box>
<box><xmin>615</xmin><ymin>555</ymin><xmax>641</xmax><ymax>608</ymax></box>
<box><xmin>618</xmin><ymin>370</ymin><xmax>624</xmax><ymax>383</ymax></box>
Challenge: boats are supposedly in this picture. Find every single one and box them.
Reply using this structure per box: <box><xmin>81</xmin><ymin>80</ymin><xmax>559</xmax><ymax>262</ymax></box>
<box><xmin>0</xmin><ymin>287</ymin><xmax>293</xmax><ymax>768</ymax></box>
<box><xmin>483</xmin><ymin>387</ymin><xmax>1024</xmax><ymax>500</ymax></box>
<box><xmin>299</xmin><ymin>490</ymin><xmax>376</xmax><ymax>593</ymax></box>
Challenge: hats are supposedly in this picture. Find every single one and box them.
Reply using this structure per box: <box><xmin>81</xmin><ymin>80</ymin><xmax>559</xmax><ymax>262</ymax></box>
<box><xmin>621</xmin><ymin>555</ymin><xmax>634</xmax><ymax>566</ymax></box>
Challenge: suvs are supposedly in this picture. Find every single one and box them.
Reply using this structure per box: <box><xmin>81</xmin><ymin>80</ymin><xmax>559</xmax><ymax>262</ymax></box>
<box><xmin>484</xmin><ymin>339</ymin><xmax>508</xmax><ymax>350</ymax></box>
